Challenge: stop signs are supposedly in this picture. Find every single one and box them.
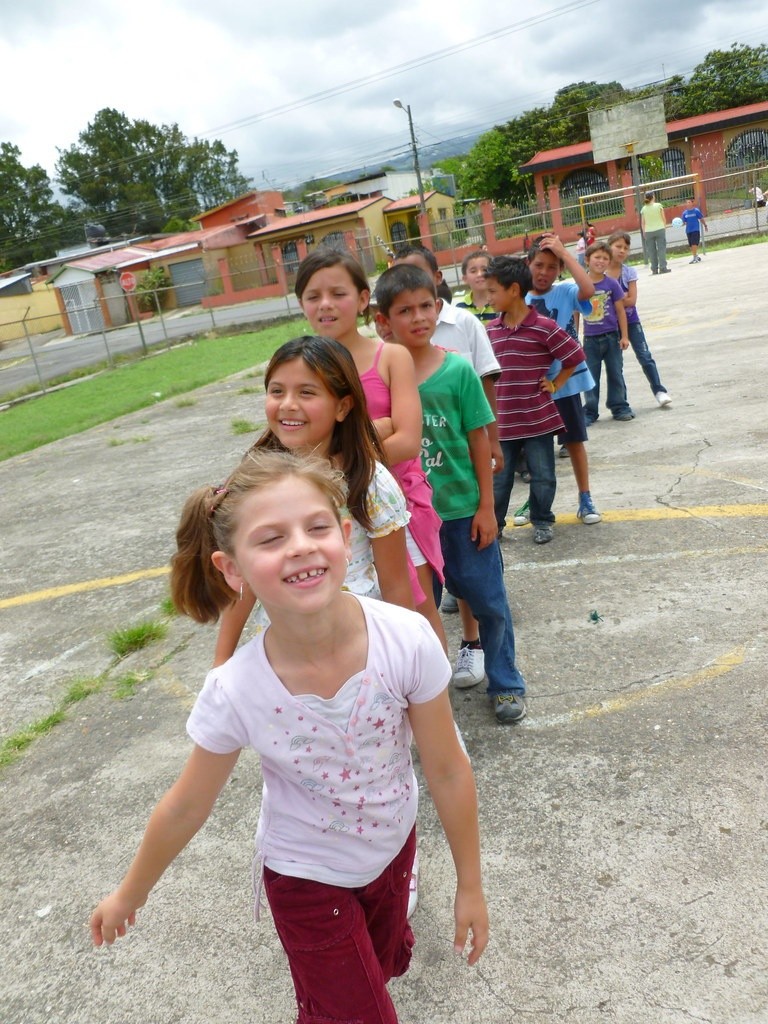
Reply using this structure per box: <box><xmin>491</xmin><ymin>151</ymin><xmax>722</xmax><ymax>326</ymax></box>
<box><xmin>118</xmin><ymin>272</ymin><xmax>136</xmax><ymax>292</ymax></box>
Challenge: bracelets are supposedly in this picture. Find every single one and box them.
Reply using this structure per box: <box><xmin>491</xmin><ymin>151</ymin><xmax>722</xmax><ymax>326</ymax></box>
<box><xmin>705</xmin><ymin>225</ymin><xmax>707</xmax><ymax>227</ymax></box>
<box><xmin>551</xmin><ymin>380</ymin><xmax>556</xmax><ymax>394</ymax></box>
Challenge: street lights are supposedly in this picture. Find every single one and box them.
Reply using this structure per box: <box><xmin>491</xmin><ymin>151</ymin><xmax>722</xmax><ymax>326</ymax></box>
<box><xmin>392</xmin><ymin>100</ymin><xmax>427</xmax><ymax>213</ymax></box>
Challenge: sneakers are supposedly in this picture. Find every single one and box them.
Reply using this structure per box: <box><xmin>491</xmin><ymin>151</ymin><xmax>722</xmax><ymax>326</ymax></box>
<box><xmin>494</xmin><ymin>693</ymin><xmax>525</xmax><ymax>723</ymax></box>
<box><xmin>454</xmin><ymin>643</ymin><xmax>486</xmax><ymax>688</ymax></box>
<box><xmin>442</xmin><ymin>591</ymin><xmax>458</xmax><ymax>612</ymax></box>
<box><xmin>577</xmin><ymin>491</ymin><xmax>601</xmax><ymax>524</ymax></box>
<box><xmin>655</xmin><ymin>390</ymin><xmax>672</xmax><ymax>406</ymax></box>
<box><xmin>514</xmin><ymin>499</ymin><xmax>530</xmax><ymax>526</ymax></box>
<box><xmin>406</xmin><ymin>849</ymin><xmax>420</xmax><ymax>918</ymax></box>
<box><xmin>534</xmin><ymin>523</ymin><xmax>552</xmax><ymax>543</ymax></box>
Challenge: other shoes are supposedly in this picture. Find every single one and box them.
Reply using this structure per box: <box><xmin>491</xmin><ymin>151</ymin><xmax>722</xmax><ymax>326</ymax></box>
<box><xmin>653</xmin><ymin>269</ymin><xmax>658</xmax><ymax>274</ymax></box>
<box><xmin>689</xmin><ymin>255</ymin><xmax>701</xmax><ymax>264</ymax></box>
<box><xmin>659</xmin><ymin>267</ymin><xmax>671</xmax><ymax>274</ymax></box>
<box><xmin>585</xmin><ymin>416</ymin><xmax>597</xmax><ymax>426</ymax></box>
<box><xmin>521</xmin><ymin>471</ymin><xmax>531</xmax><ymax>482</ymax></box>
<box><xmin>452</xmin><ymin>720</ymin><xmax>471</xmax><ymax>767</ymax></box>
<box><xmin>559</xmin><ymin>444</ymin><xmax>569</xmax><ymax>457</ymax></box>
<box><xmin>616</xmin><ymin>413</ymin><xmax>632</xmax><ymax>421</ymax></box>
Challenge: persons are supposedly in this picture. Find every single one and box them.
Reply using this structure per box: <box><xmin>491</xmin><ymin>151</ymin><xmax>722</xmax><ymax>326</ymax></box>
<box><xmin>574</xmin><ymin>243</ymin><xmax>633</xmax><ymax>426</ymax></box>
<box><xmin>603</xmin><ymin>233</ymin><xmax>672</xmax><ymax>407</ymax></box>
<box><xmin>682</xmin><ymin>199</ymin><xmax>708</xmax><ymax>264</ymax></box>
<box><xmin>574</xmin><ymin>222</ymin><xmax>595</xmax><ymax>269</ymax></box>
<box><xmin>749</xmin><ymin>186</ymin><xmax>768</xmax><ymax>207</ymax></box>
<box><xmin>357</xmin><ymin>233</ymin><xmax>599</xmax><ymax>685</ymax></box>
<box><xmin>91</xmin><ymin>454</ymin><xmax>488</xmax><ymax>1024</ymax></box>
<box><xmin>211</xmin><ymin>336</ymin><xmax>418</xmax><ymax>917</ymax></box>
<box><xmin>377</xmin><ymin>262</ymin><xmax>529</xmax><ymax>723</ymax></box>
<box><xmin>480</xmin><ymin>243</ymin><xmax>488</xmax><ymax>251</ymax></box>
<box><xmin>296</xmin><ymin>249</ymin><xmax>467</xmax><ymax>756</ymax></box>
<box><xmin>641</xmin><ymin>192</ymin><xmax>671</xmax><ymax>275</ymax></box>
